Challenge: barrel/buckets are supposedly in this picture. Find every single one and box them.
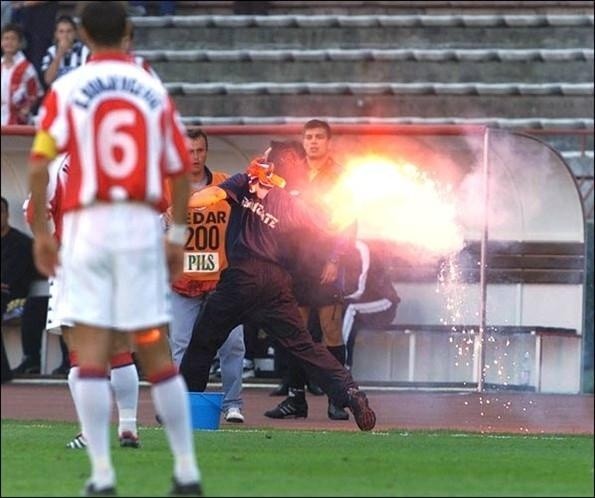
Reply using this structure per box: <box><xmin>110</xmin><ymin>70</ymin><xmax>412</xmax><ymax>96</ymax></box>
<box><xmin>189</xmin><ymin>390</ymin><xmax>229</xmax><ymax>430</ymax></box>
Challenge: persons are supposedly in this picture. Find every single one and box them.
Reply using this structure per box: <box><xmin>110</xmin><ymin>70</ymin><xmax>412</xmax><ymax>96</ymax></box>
<box><xmin>1</xmin><ymin>0</ymin><xmax>177</xmax><ymax>125</ymax></box>
<box><xmin>0</xmin><ymin>198</ymin><xmax>33</xmax><ymax>379</ymax></box>
<box><xmin>27</xmin><ymin>0</ymin><xmax>206</xmax><ymax>496</ymax></box>
<box><xmin>21</xmin><ymin>153</ymin><xmax>173</xmax><ymax>450</ymax></box>
<box><xmin>12</xmin><ymin>278</ymin><xmax>69</xmax><ymax>378</ymax></box>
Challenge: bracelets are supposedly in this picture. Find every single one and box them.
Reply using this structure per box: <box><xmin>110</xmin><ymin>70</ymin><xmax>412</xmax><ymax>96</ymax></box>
<box><xmin>167</xmin><ymin>223</ymin><xmax>187</xmax><ymax>247</ymax></box>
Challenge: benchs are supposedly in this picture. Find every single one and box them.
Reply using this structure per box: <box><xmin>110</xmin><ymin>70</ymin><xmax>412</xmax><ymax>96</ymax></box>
<box><xmin>345</xmin><ymin>236</ymin><xmax>586</xmax><ymax>394</ymax></box>
<box><xmin>130</xmin><ymin>2</ymin><xmax>594</xmax><ymax>164</ymax></box>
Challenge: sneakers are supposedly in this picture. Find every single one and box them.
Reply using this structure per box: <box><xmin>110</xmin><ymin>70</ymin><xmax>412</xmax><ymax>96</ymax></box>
<box><xmin>51</xmin><ymin>364</ymin><xmax>72</xmax><ymax>379</ymax></box>
<box><xmin>347</xmin><ymin>391</ymin><xmax>376</xmax><ymax>431</ymax></box>
<box><xmin>269</xmin><ymin>381</ymin><xmax>290</xmax><ymax>397</ymax></box>
<box><xmin>64</xmin><ymin>432</ymin><xmax>88</xmax><ymax>450</ymax></box>
<box><xmin>327</xmin><ymin>397</ymin><xmax>349</xmax><ymax>420</ymax></box>
<box><xmin>11</xmin><ymin>355</ymin><xmax>41</xmax><ymax>379</ymax></box>
<box><xmin>263</xmin><ymin>397</ymin><xmax>308</xmax><ymax>419</ymax></box>
<box><xmin>223</xmin><ymin>407</ymin><xmax>245</xmax><ymax>423</ymax></box>
<box><xmin>307</xmin><ymin>383</ymin><xmax>324</xmax><ymax>396</ymax></box>
<box><xmin>117</xmin><ymin>431</ymin><xmax>140</xmax><ymax>448</ymax></box>
